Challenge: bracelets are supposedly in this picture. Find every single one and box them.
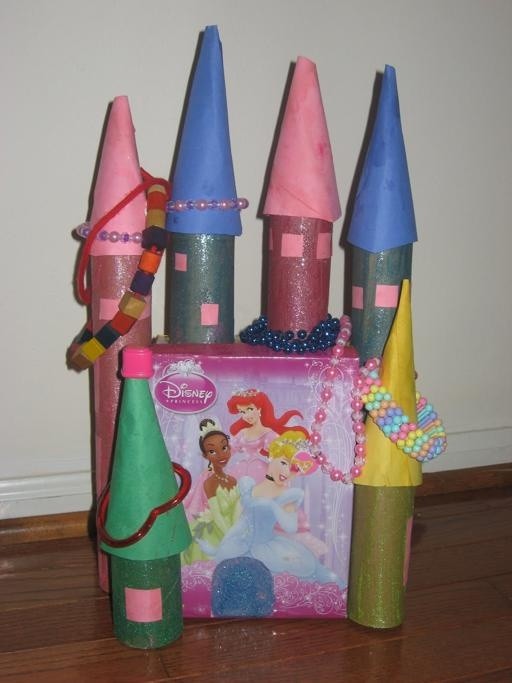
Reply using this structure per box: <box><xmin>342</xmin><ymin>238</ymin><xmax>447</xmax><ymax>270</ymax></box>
<box><xmin>75</xmin><ymin>222</ymin><xmax>141</xmax><ymax>244</ymax></box>
<box><xmin>363</xmin><ymin>384</ymin><xmax>446</xmax><ymax>461</ymax></box>
<box><xmin>92</xmin><ymin>461</ymin><xmax>192</xmax><ymax>547</ymax></box>
<box><xmin>67</xmin><ymin>175</ymin><xmax>171</xmax><ymax>372</ymax></box>
<box><xmin>163</xmin><ymin>200</ymin><xmax>247</xmax><ymax>212</ymax></box>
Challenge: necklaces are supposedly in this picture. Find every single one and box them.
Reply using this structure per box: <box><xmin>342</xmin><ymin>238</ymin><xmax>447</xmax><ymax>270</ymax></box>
<box><xmin>310</xmin><ymin>316</ymin><xmax>384</xmax><ymax>484</ymax></box>
<box><xmin>241</xmin><ymin>316</ymin><xmax>338</xmax><ymax>354</ymax></box>
